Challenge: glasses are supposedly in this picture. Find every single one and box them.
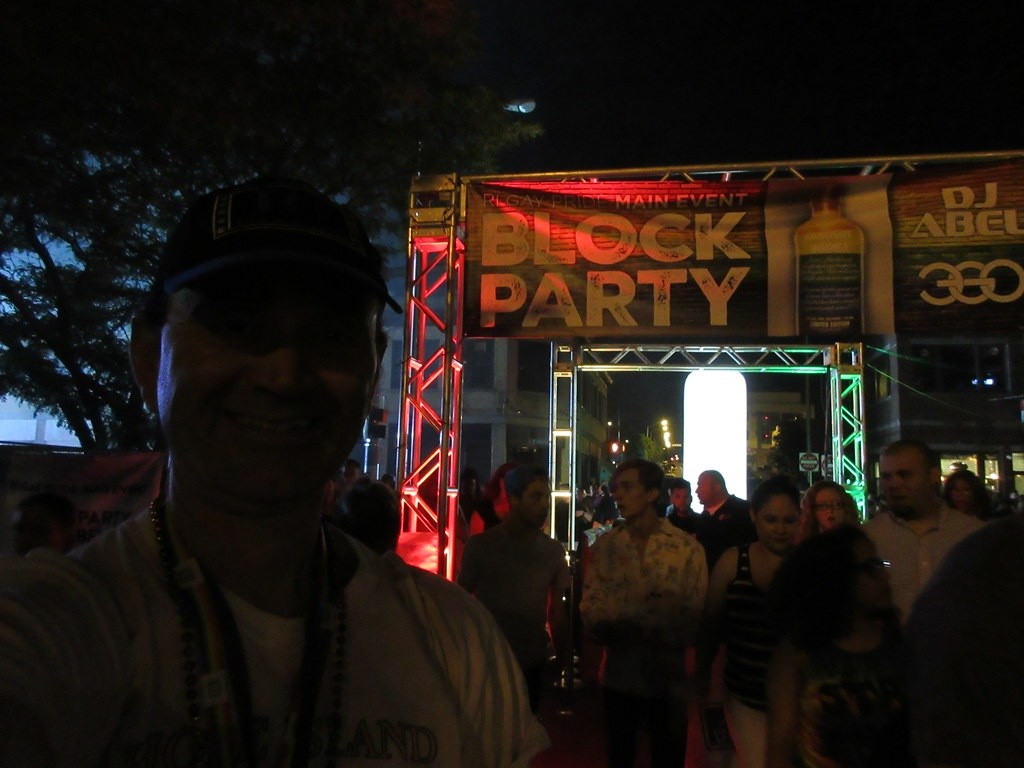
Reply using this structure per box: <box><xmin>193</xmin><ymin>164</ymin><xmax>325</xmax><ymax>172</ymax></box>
<box><xmin>811</xmin><ymin>504</ymin><xmax>847</xmax><ymax>512</ymax></box>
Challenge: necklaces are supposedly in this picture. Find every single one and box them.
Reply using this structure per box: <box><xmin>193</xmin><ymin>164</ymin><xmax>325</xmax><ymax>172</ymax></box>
<box><xmin>148</xmin><ymin>500</ymin><xmax>348</xmax><ymax>767</ymax></box>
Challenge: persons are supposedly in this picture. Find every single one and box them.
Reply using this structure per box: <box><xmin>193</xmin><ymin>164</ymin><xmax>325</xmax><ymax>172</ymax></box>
<box><xmin>580</xmin><ymin>459</ymin><xmax>709</xmax><ymax>768</ymax></box>
<box><xmin>692</xmin><ymin>439</ymin><xmax>1024</xmax><ymax>768</ymax></box>
<box><xmin>321</xmin><ymin>457</ymin><xmax>519</xmax><ymax>586</ymax></box>
<box><xmin>457</xmin><ymin>463</ymin><xmax>569</xmax><ymax>714</ymax></box>
<box><xmin>0</xmin><ymin>174</ymin><xmax>552</xmax><ymax>768</ymax></box>
<box><xmin>575</xmin><ymin>475</ymin><xmax>760</xmax><ymax>579</ymax></box>
<box><xmin>11</xmin><ymin>493</ymin><xmax>75</xmax><ymax>553</ymax></box>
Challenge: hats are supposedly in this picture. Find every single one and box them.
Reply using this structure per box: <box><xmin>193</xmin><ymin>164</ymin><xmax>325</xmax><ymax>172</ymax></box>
<box><xmin>160</xmin><ymin>177</ymin><xmax>404</xmax><ymax>318</ymax></box>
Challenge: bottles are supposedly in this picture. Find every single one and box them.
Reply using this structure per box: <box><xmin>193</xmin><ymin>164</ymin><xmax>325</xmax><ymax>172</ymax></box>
<box><xmin>792</xmin><ymin>183</ymin><xmax>866</xmax><ymax>335</ymax></box>
